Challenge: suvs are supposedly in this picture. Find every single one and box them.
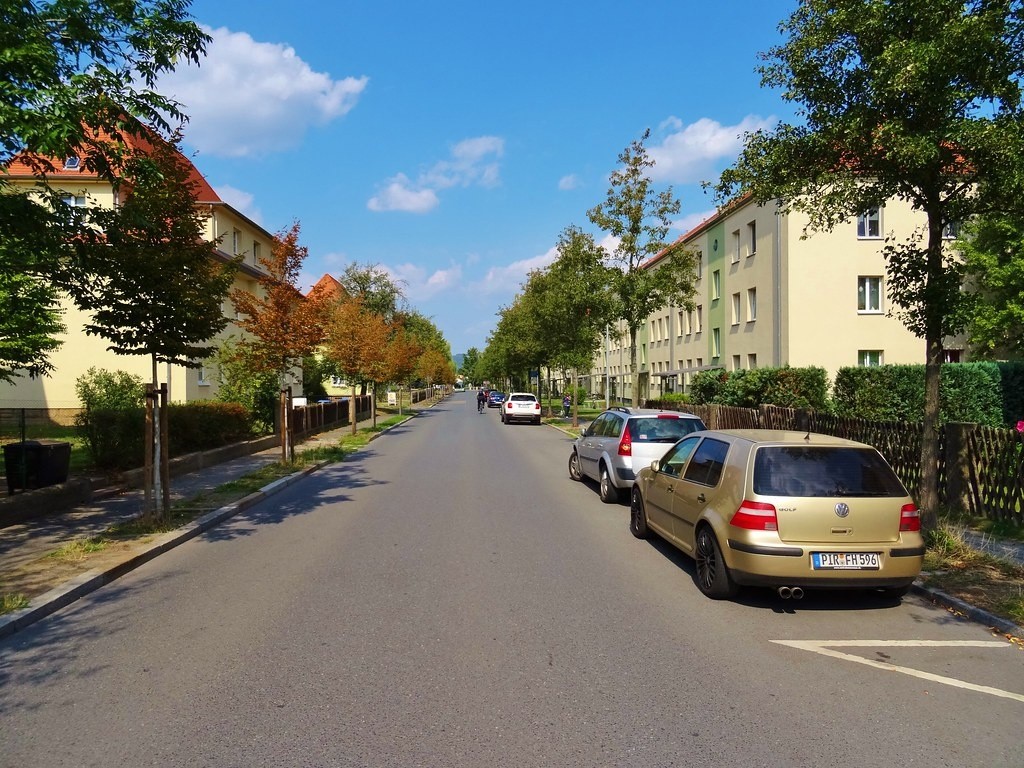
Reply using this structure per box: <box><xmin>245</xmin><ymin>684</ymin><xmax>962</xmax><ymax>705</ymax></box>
<box><xmin>568</xmin><ymin>406</ymin><xmax>711</xmax><ymax>503</ymax></box>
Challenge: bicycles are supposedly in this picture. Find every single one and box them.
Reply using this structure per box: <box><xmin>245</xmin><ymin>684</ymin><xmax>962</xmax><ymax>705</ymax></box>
<box><xmin>479</xmin><ymin>399</ymin><xmax>484</xmax><ymax>414</ymax></box>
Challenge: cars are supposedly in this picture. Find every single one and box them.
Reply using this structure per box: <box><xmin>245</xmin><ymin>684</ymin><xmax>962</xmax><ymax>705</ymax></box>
<box><xmin>487</xmin><ymin>391</ymin><xmax>507</xmax><ymax>408</ymax></box>
<box><xmin>498</xmin><ymin>392</ymin><xmax>541</xmax><ymax>425</ymax></box>
<box><xmin>483</xmin><ymin>389</ymin><xmax>497</xmax><ymax>402</ymax></box>
<box><xmin>630</xmin><ymin>427</ymin><xmax>927</xmax><ymax>601</ymax></box>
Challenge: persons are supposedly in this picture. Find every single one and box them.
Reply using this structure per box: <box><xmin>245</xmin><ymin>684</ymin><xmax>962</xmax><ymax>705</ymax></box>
<box><xmin>477</xmin><ymin>391</ymin><xmax>485</xmax><ymax>410</ymax></box>
<box><xmin>562</xmin><ymin>393</ymin><xmax>571</xmax><ymax>418</ymax></box>
